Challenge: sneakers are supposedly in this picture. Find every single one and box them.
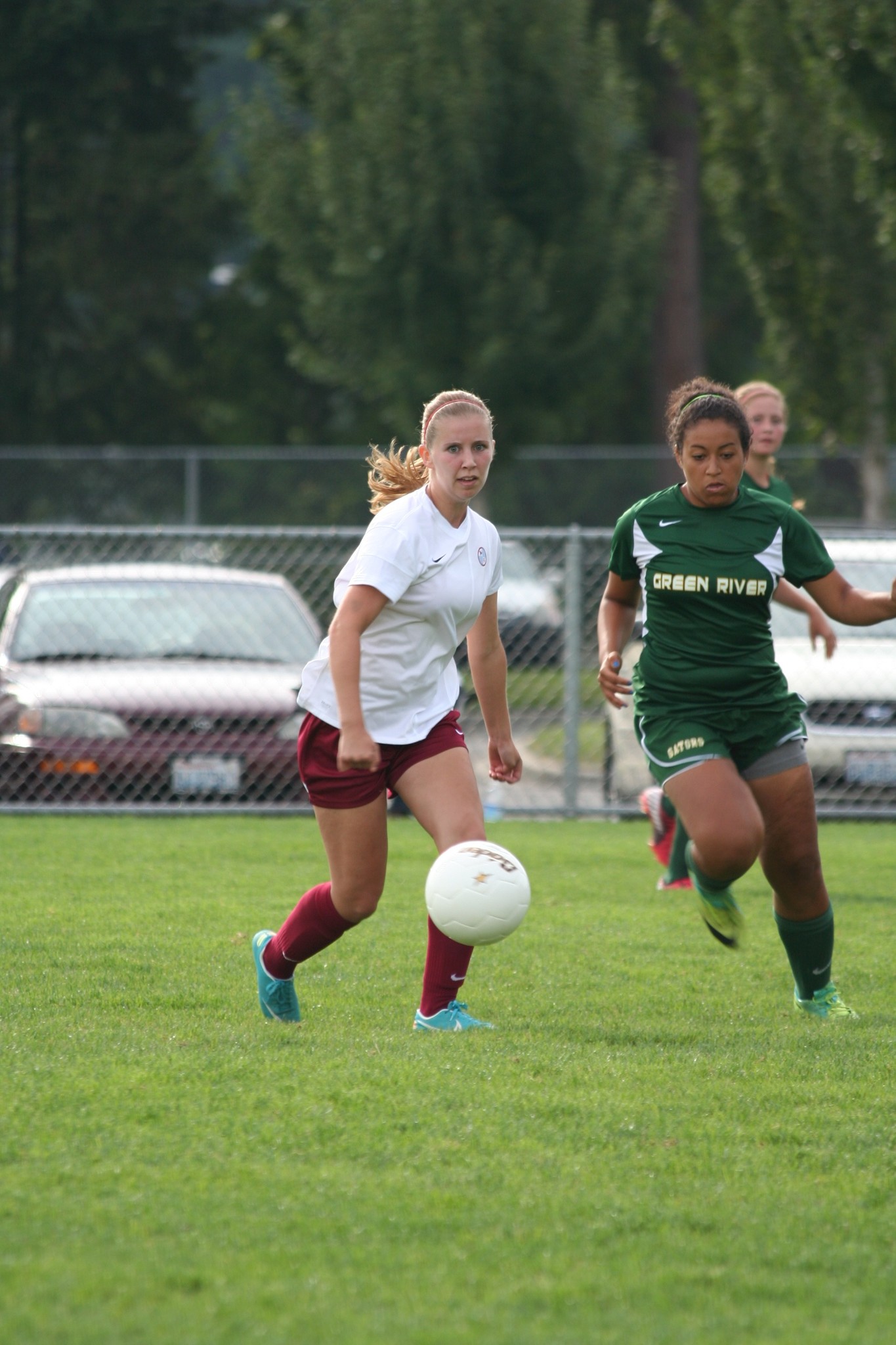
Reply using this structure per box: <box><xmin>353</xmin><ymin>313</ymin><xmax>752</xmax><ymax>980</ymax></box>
<box><xmin>793</xmin><ymin>982</ymin><xmax>858</xmax><ymax>1019</ymax></box>
<box><xmin>685</xmin><ymin>841</ymin><xmax>743</xmax><ymax>946</ymax></box>
<box><xmin>640</xmin><ymin>786</ymin><xmax>676</xmax><ymax>867</ymax></box>
<box><xmin>414</xmin><ymin>1001</ymin><xmax>491</xmax><ymax>1032</ymax></box>
<box><xmin>252</xmin><ymin>931</ymin><xmax>300</xmax><ymax>1022</ymax></box>
<box><xmin>657</xmin><ymin>876</ymin><xmax>692</xmax><ymax>890</ymax></box>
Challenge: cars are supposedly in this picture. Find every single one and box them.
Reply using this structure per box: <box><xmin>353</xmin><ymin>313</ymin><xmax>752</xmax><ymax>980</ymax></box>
<box><xmin>0</xmin><ymin>564</ymin><xmax>324</xmax><ymax>805</ymax></box>
<box><xmin>455</xmin><ymin>539</ymin><xmax>566</xmax><ymax>666</ymax></box>
<box><xmin>604</xmin><ymin>538</ymin><xmax>896</xmax><ymax>802</ymax></box>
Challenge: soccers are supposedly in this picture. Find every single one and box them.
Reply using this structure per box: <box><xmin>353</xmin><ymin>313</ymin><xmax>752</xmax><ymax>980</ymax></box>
<box><xmin>426</xmin><ymin>840</ymin><xmax>532</xmax><ymax>947</ymax></box>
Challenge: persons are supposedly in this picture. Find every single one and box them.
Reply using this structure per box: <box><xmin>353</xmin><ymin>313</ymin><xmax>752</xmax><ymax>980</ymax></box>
<box><xmin>250</xmin><ymin>394</ymin><xmax>521</xmax><ymax>1032</ymax></box>
<box><xmin>598</xmin><ymin>378</ymin><xmax>896</xmax><ymax>1026</ymax></box>
